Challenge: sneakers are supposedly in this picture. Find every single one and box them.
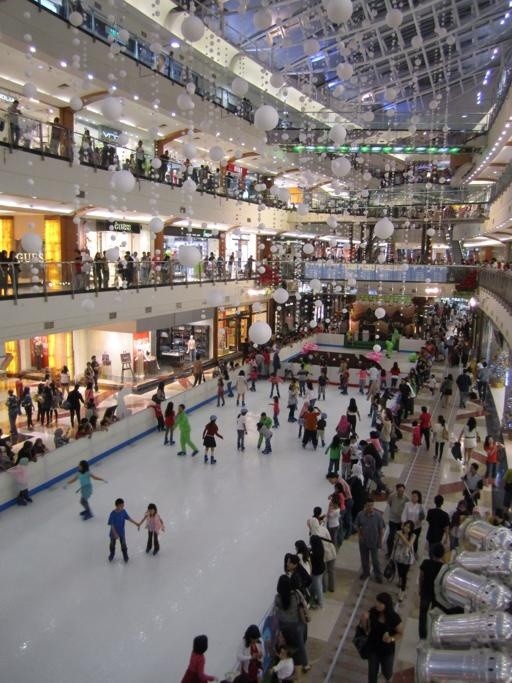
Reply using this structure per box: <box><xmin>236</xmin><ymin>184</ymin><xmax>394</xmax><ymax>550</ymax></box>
<box><xmin>372</xmin><ymin>576</ymin><xmax>382</xmax><ymax>583</ymax></box>
<box><xmin>272</xmin><ymin>425</ymin><xmax>278</xmax><ymax>428</ymax></box>
<box><xmin>192</xmin><ymin>450</ymin><xmax>198</xmax><ymax>456</ymax></box>
<box><xmin>146</xmin><ymin>547</ymin><xmax>151</xmax><ymax>552</ymax></box>
<box><xmin>397</xmin><ymin>578</ymin><xmax>403</xmax><ymax>587</ymax></box>
<box><xmin>288</xmin><ymin>416</ymin><xmax>297</xmax><ymax>422</ymax></box>
<box><xmin>153</xmin><ymin>549</ymin><xmax>157</xmax><ymax>554</ymax></box>
<box><xmin>80</xmin><ymin>510</ymin><xmax>92</xmax><ymax>520</ymax></box>
<box><xmin>360</xmin><ymin>574</ymin><xmax>368</xmax><ymax>580</ymax></box>
<box><xmin>124</xmin><ymin>554</ymin><xmax>128</xmax><ymax>561</ymax></box>
<box><xmin>177</xmin><ymin>452</ymin><xmax>186</xmax><ymax>455</ymax></box>
<box><xmin>109</xmin><ymin>553</ymin><xmax>114</xmax><ymax>560</ymax></box>
<box><xmin>164</xmin><ymin>441</ymin><xmax>175</xmax><ymax>445</ymax></box>
<box><xmin>204</xmin><ymin>455</ymin><xmax>216</xmax><ymax>464</ymax></box>
<box><xmin>302</xmin><ymin>664</ymin><xmax>311</xmax><ymax>673</ymax></box>
<box><xmin>262</xmin><ymin>448</ymin><xmax>271</xmax><ymax>454</ymax></box>
<box><xmin>399</xmin><ymin>591</ymin><xmax>405</xmax><ymax>602</ymax></box>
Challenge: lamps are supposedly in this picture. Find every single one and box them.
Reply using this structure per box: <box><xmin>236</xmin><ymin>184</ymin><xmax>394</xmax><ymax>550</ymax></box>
<box><xmin>411</xmin><ymin>512</ymin><xmax>512</xmax><ymax>682</ymax></box>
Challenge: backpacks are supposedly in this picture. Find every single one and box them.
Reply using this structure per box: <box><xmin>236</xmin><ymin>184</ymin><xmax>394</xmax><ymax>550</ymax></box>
<box><xmin>10</xmin><ymin>396</ymin><xmax>20</xmax><ymax>414</ymax></box>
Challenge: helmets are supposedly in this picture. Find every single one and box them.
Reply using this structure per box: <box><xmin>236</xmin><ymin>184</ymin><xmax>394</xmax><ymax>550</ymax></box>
<box><xmin>210</xmin><ymin>415</ymin><xmax>216</xmax><ymax>421</ymax></box>
<box><xmin>241</xmin><ymin>409</ymin><xmax>247</xmax><ymax>413</ymax></box>
<box><xmin>321</xmin><ymin>413</ymin><xmax>327</xmax><ymax>418</ymax></box>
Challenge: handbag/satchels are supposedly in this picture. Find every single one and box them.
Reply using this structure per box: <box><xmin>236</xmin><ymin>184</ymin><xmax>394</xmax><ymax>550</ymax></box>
<box><xmin>384</xmin><ymin>560</ymin><xmax>396</xmax><ymax>579</ymax></box>
<box><xmin>418</xmin><ymin>511</ymin><xmax>425</xmax><ymax>520</ymax></box>
<box><xmin>442</xmin><ymin>429</ymin><xmax>449</xmax><ymax>440</ymax></box>
<box><xmin>352</xmin><ymin>625</ymin><xmax>371</xmax><ymax>658</ymax></box>
<box><xmin>396</xmin><ymin>429</ymin><xmax>403</xmax><ymax>439</ymax></box>
<box><xmin>470</xmin><ymin>391</ymin><xmax>477</xmax><ymax>399</ymax></box>
<box><xmin>22</xmin><ymin>401</ymin><xmax>31</xmax><ymax>407</ymax></box>
<box><xmin>445</xmin><ymin>389</ymin><xmax>452</xmax><ymax>395</ymax></box>
<box><xmin>62</xmin><ymin>399</ymin><xmax>72</xmax><ymax>410</ymax></box>
<box><xmin>298</xmin><ymin>601</ymin><xmax>311</xmax><ymax>623</ymax></box>
<box><xmin>180</xmin><ymin>669</ymin><xmax>208</xmax><ymax>683</ymax></box>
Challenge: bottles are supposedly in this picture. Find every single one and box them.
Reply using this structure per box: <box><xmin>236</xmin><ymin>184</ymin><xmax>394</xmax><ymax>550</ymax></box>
<box><xmin>382</xmin><ymin>632</ymin><xmax>389</xmax><ymax>641</ymax></box>
